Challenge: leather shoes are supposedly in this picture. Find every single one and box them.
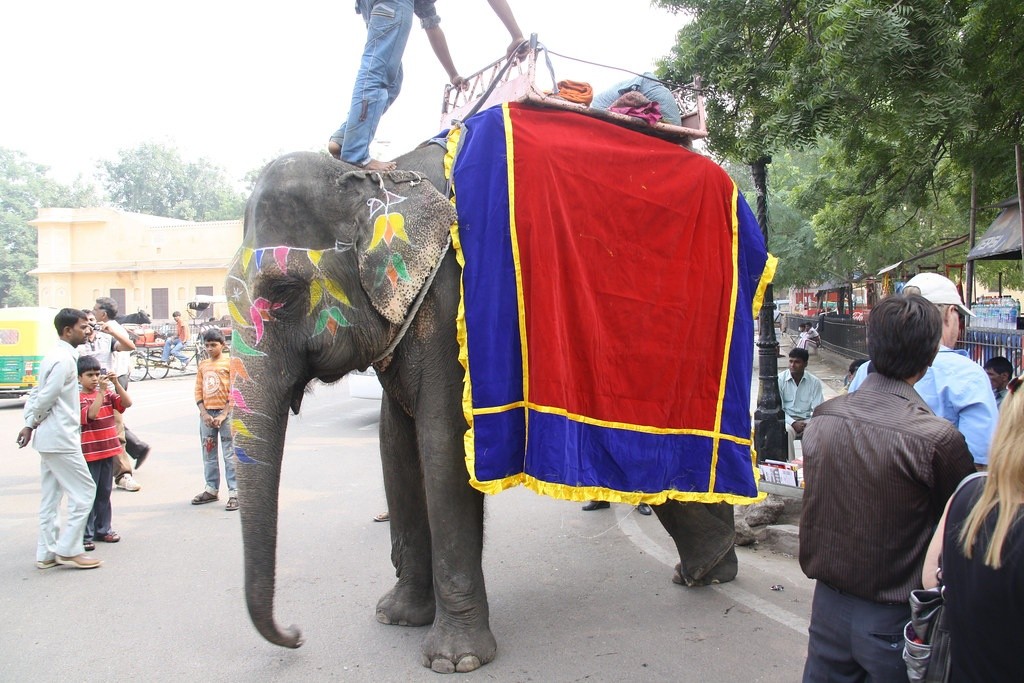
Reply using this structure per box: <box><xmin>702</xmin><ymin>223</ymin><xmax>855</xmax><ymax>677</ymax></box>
<box><xmin>635</xmin><ymin>504</ymin><xmax>652</xmax><ymax>516</ymax></box>
<box><xmin>582</xmin><ymin>500</ymin><xmax>610</xmax><ymax>510</ymax></box>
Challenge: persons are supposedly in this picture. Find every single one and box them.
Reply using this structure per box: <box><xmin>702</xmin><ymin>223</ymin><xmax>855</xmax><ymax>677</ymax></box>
<box><xmin>16</xmin><ymin>309</ymin><xmax>104</xmax><ymax>569</ymax></box>
<box><xmin>161</xmin><ymin>311</ymin><xmax>190</xmax><ymax>368</ymax></box>
<box><xmin>77</xmin><ymin>355</ymin><xmax>132</xmax><ymax>551</ymax></box>
<box><xmin>800</xmin><ymin>271</ymin><xmax>1024</xmax><ymax>683</ymax></box>
<box><xmin>796</xmin><ymin>322</ymin><xmax>821</xmax><ymax>349</ymax></box>
<box><xmin>192</xmin><ymin>328</ymin><xmax>240</xmax><ymax>510</ymax></box>
<box><xmin>328</xmin><ymin>0</ymin><xmax>529</xmax><ymax>171</ymax></box>
<box><xmin>777</xmin><ymin>348</ymin><xmax>824</xmax><ymax>462</ymax></box>
<box><xmin>78</xmin><ymin>297</ymin><xmax>151</xmax><ymax>491</ymax></box>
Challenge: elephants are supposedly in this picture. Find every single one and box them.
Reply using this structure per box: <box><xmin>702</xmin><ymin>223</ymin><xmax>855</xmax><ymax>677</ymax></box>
<box><xmin>224</xmin><ymin>101</ymin><xmax>739</xmax><ymax>674</ymax></box>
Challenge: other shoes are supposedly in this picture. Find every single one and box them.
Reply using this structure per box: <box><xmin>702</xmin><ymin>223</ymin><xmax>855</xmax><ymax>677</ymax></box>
<box><xmin>91</xmin><ymin>531</ymin><xmax>120</xmax><ymax>543</ymax></box>
<box><xmin>135</xmin><ymin>445</ymin><xmax>150</xmax><ymax>469</ymax></box>
<box><xmin>373</xmin><ymin>511</ymin><xmax>390</xmax><ymax>521</ymax></box>
<box><xmin>37</xmin><ymin>554</ymin><xmax>104</xmax><ymax>568</ymax></box>
<box><xmin>83</xmin><ymin>543</ymin><xmax>95</xmax><ymax>551</ymax></box>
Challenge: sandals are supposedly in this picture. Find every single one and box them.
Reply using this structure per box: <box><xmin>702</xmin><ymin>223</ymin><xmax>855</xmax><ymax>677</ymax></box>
<box><xmin>191</xmin><ymin>491</ymin><xmax>219</xmax><ymax>504</ymax></box>
<box><xmin>225</xmin><ymin>497</ymin><xmax>240</xmax><ymax>510</ymax></box>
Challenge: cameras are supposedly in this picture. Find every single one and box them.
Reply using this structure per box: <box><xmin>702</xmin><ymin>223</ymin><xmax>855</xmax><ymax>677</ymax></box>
<box><xmin>91</xmin><ymin>324</ymin><xmax>100</xmax><ymax>330</ymax></box>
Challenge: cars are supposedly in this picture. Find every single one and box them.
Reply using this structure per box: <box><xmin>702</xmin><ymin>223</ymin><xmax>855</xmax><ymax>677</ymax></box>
<box><xmin>772</xmin><ymin>300</ymin><xmax>789</xmax><ymax>324</ymax></box>
<box><xmin>214</xmin><ymin>320</ymin><xmax>233</xmax><ymax>339</ymax></box>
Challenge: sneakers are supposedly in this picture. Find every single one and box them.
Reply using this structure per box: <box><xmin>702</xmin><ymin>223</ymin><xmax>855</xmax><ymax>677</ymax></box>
<box><xmin>115</xmin><ymin>474</ymin><xmax>140</xmax><ymax>491</ymax></box>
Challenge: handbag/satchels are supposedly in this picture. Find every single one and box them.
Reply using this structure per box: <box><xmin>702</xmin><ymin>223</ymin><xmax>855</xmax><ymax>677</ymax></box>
<box><xmin>902</xmin><ymin>472</ymin><xmax>989</xmax><ymax>683</ymax></box>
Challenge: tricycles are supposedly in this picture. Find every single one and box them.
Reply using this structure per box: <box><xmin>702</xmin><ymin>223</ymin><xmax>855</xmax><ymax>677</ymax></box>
<box><xmin>127</xmin><ymin>335</ymin><xmax>208</xmax><ymax>382</ymax></box>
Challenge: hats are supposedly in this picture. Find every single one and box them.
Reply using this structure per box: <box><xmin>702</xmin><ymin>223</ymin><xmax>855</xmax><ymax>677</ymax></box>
<box><xmin>901</xmin><ymin>273</ymin><xmax>976</xmax><ymax>317</ymax></box>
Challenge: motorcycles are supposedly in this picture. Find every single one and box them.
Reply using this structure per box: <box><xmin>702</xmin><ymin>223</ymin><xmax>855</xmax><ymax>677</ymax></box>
<box><xmin>0</xmin><ymin>308</ymin><xmax>63</xmax><ymax>398</ymax></box>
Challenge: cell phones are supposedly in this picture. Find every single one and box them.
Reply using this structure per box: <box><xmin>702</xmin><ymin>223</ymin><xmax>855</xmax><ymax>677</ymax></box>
<box><xmin>100</xmin><ymin>368</ymin><xmax>107</xmax><ymax>380</ymax></box>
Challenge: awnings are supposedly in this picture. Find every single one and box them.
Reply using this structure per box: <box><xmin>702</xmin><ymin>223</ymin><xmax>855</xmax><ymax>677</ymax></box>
<box><xmin>966</xmin><ymin>195</ymin><xmax>1024</xmax><ymax>261</ymax></box>
